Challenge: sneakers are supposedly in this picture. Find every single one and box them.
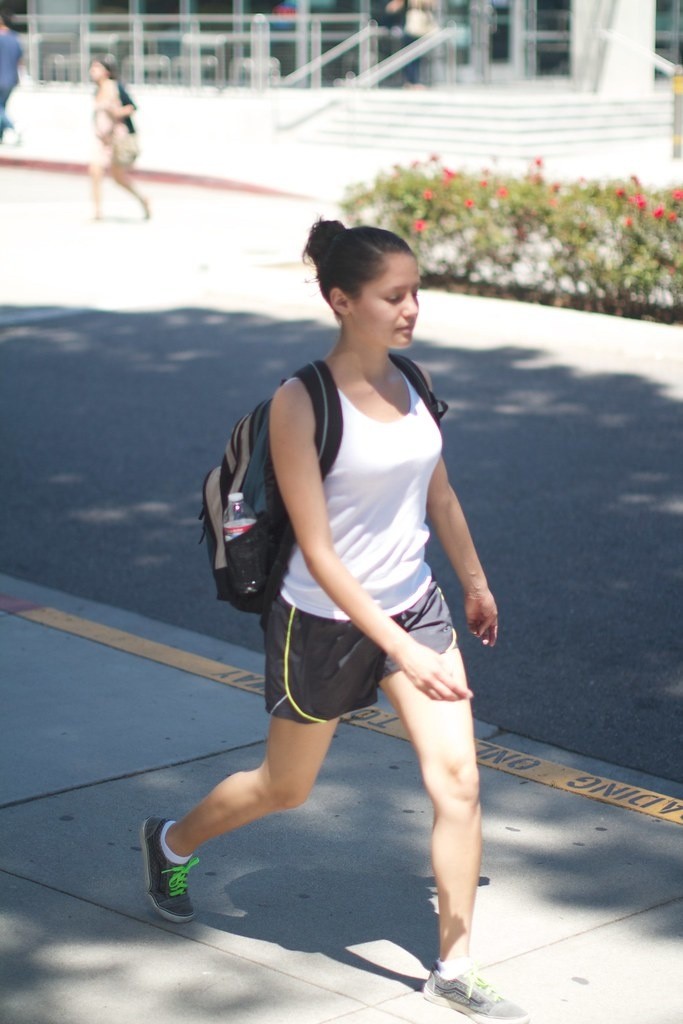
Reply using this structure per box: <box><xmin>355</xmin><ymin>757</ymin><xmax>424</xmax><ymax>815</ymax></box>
<box><xmin>422</xmin><ymin>966</ymin><xmax>530</xmax><ymax>1024</ymax></box>
<box><xmin>140</xmin><ymin>815</ymin><xmax>200</xmax><ymax>923</ymax></box>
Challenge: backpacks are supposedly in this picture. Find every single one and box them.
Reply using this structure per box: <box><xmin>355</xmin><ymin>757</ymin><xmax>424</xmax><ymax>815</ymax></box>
<box><xmin>197</xmin><ymin>354</ymin><xmax>448</xmax><ymax>614</ymax></box>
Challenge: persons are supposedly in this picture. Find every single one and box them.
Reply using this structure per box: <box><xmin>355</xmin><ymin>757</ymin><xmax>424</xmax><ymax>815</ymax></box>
<box><xmin>0</xmin><ymin>9</ymin><xmax>23</xmax><ymax>144</ymax></box>
<box><xmin>85</xmin><ymin>59</ymin><xmax>152</xmax><ymax>221</ymax></box>
<box><xmin>136</xmin><ymin>219</ymin><xmax>529</xmax><ymax>1024</ymax></box>
<box><xmin>385</xmin><ymin>0</ymin><xmax>429</xmax><ymax>86</ymax></box>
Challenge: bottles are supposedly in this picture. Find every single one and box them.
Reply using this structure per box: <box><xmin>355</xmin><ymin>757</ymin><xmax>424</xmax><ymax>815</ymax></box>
<box><xmin>224</xmin><ymin>492</ymin><xmax>263</xmax><ymax>595</ymax></box>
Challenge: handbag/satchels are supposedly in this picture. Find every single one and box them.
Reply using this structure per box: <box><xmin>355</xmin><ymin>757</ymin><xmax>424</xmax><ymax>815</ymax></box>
<box><xmin>113</xmin><ymin>132</ymin><xmax>139</xmax><ymax>165</ymax></box>
